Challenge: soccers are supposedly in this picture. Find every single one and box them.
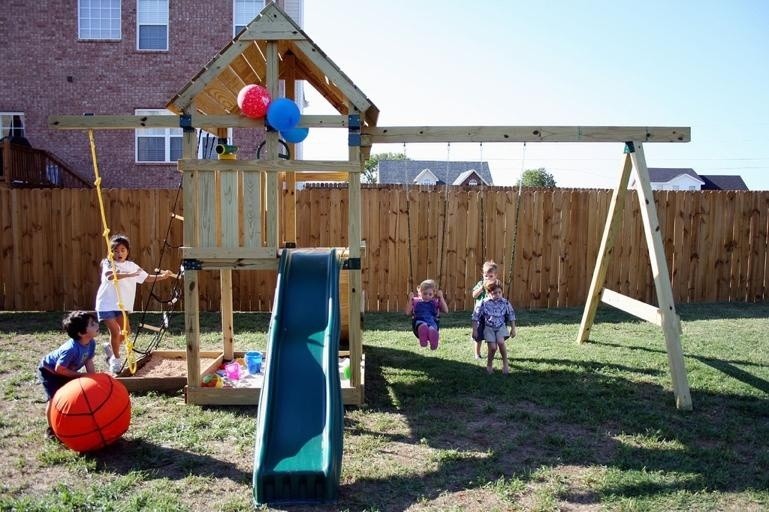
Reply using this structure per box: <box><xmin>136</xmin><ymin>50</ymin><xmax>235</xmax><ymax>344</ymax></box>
<box><xmin>202</xmin><ymin>374</ymin><xmax>223</xmax><ymax>387</ymax></box>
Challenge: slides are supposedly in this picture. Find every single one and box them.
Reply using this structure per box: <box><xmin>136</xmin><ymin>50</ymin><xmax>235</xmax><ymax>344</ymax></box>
<box><xmin>252</xmin><ymin>246</ymin><xmax>344</xmax><ymax>503</ymax></box>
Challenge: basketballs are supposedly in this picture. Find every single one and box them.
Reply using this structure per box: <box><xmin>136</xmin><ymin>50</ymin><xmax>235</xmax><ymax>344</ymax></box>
<box><xmin>47</xmin><ymin>373</ymin><xmax>131</xmax><ymax>453</ymax></box>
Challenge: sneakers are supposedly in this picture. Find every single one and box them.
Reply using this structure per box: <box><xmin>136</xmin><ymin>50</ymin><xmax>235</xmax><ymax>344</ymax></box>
<box><xmin>418</xmin><ymin>324</ymin><xmax>438</xmax><ymax>350</ymax></box>
<box><xmin>487</xmin><ymin>365</ymin><xmax>493</xmax><ymax>375</ymax></box>
<box><xmin>102</xmin><ymin>342</ymin><xmax>121</xmax><ymax>376</ymax></box>
<box><xmin>502</xmin><ymin>365</ymin><xmax>509</xmax><ymax>375</ymax></box>
<box><xmin>474</xmin><ymin>354</ymin><xmax>481</xmax><ymax>360</ymax></box>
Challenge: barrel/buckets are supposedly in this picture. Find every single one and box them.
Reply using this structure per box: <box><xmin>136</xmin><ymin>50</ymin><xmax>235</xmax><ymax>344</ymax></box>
<box><xmin>244</xmin><ymin>351</ymin><xmax>264</xmax><ymax>374</ymax></box>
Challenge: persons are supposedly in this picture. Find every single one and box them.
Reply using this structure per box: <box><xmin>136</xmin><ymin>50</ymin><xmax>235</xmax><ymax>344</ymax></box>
<box><xmin>470</xmin><ymin>278</ymin><xmax>516</xmax><ymax>377</ymax></box>
<box><xmin>405</xmin><ymin>278</ymin><xmax>450</xmax><ymax>351</ymax></box>
<box><xmin>94</xmin><ymin>234</ymin><xmax>173</xmax><ymax>374</ymax></box>
<box><xmin>37</xmin><ymin>308</ymin><xmax>99</xmax><ymax>441</ymax></box>
<box><xmin>471</xmin><ymin>259</ymin><xmax>499</xmax><ymax>360</ymax></box>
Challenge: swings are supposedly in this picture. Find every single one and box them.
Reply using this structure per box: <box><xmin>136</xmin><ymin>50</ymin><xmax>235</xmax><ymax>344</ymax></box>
<box><xmin>479</xmin><ymin>141</ymin><xmax>527</xmax><ymax>339</ymax></box>
<box><xmin>404</xmin><ymin>142</ymin><xmax>452</xmax><ymax>338</ymax></box>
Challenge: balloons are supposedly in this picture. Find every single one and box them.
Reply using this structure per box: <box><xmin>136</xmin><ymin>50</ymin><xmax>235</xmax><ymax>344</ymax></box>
<box><xmin>237</xmin><ymin>84</ymin><xmax>270</xmax><ymax>119</ymax></box>
<box><xmin>279</xmin><ymin>127</ymin><xmax>310</xmax><ymax>144</ymax></box>
<box><xmin>265</xmin><ymin>97</ymin><xmax>301</xmax><ymax>133</ymax></box>
